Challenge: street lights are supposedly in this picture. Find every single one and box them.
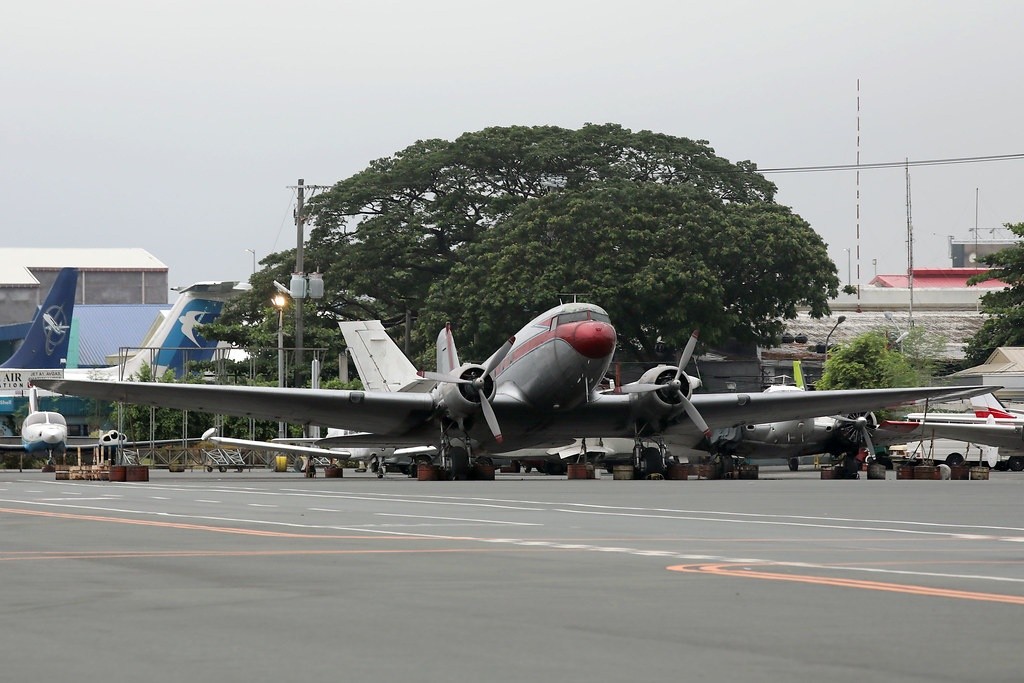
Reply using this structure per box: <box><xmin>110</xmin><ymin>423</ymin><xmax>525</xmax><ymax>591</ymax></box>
<box><xmin>824</xmin><ymin>314</ymin><xmax>846</xmax><ymax>363</ymax></box>
<box><xmin>244</xmin><ymin>248</ymin><xmax>257</xmax><ymax>275</ymax></box>
<box><xmin>843</xmin><ymin>247</ymin><xmax>851</xmax><ymax>285</ymax></box>
<box><xmin>885</xmin><ymin>313</ymin><xmax>905</xmax><ymax>360</ymax></box>
<box><xmin>274</xmin><ymin>296</ymin><xmax>287</xmax><ymax>443</ymax></box>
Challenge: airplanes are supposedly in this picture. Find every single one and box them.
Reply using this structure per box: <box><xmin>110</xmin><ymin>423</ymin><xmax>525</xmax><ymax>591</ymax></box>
<box><xmin>33</xmin><ymin>296</ymin><xmax>1005</xmax><ymax>483</ymax></box>
<box><xmin>1</xmin><ymin>411</ymin><xmax>204</xmax><ymax>468</ymax></box>
<box><xmin>209</xmin><ymin>384</ymin><xmax>1024</xmax><ymax>484</ymax></box>
